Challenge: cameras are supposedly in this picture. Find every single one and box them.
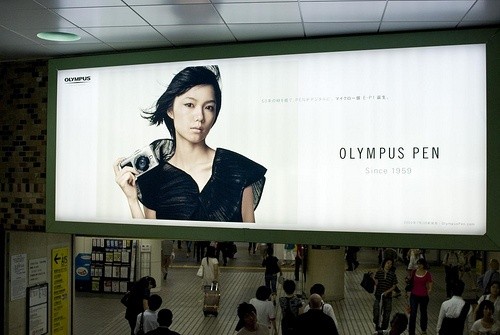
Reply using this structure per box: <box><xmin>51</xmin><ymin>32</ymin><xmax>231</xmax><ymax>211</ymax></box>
<box><xmin>120</xmin><ymin>145</ymin><xmax>159</xmax><ymax>178</ymax></box>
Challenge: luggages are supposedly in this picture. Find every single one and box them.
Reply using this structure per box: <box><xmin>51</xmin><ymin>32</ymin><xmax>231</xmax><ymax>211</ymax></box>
<box><xmin>455</xmin><ymin>271</ymin><xmax>466</xmax><ymax>297</ymax></box>
<box><xmin>203</xmin><ymin>281</ymin><xmax>220</xmax><ymax>318</ymax></box>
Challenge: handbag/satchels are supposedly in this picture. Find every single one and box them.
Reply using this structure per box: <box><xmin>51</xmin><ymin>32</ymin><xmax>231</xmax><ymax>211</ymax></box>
<box><xmin>405</xmin><ymin>269</ymin><xmax>417</xmax><ymax>291</ymax></box>
<box><xmin>170</xmin><ymin>248</ymin><xmax>176</xmax><ymax>263</ymax></box>
<box><xmin>197</xmin><ymin>265</ymin><xmax>204</xmax><ymax>278</ymax></box>
<box><xmin>360</xmin><ymin>271</ymin><xmax>376</xmax><ymax>294</ymax></box>
<box><xmin>121</xmin><ymin>291</ymin><xmax>130</xmax><ymax>306</ymax></box>
<box><xmin>137</xmin><ymin>313</ymin><xmax>144</xmax><ymax>335</ymax></box>
<box><xmin>278</xmin><ymin>273</ymin><xmax>284</xmax><ymax>284</ymax></box>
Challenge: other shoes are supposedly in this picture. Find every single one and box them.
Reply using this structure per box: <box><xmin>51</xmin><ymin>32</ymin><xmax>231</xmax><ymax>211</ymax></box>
<box><xmin>290</xmin><ymin>261</ymin><xmax>296</xmax><ymax>266</ymax></box>
<box><xmin>346</xmin><ymin>267</ymin><xmax>353</xmax><ymax>271</ymax></box>
<box><xmin>353</xmin><ymin>262</ymin><xmax>360</xmax><ymax>270</ymax></box>
<box><xmin>393</xmin><ymin>292</ymin><xmax>402</xmax><ymax>298</ymax></box>
<box><xmin>163</xmin><ymin>272</ymin><xmax>168</xmax><ymax>280</ymax></box>
<box><xmin>280</xmin><ymin>261</ymin><xmax>286</xmax><ymax>266</ymax></box>
<box><xmin>186</xmin><ymin>252</ymin><xmax>190</xmax><ymax>257</ymax></box>
<box><xmin>273</xmin><ymin>295</ymin><xmax>276</xmax><ymax>305</ymax></box>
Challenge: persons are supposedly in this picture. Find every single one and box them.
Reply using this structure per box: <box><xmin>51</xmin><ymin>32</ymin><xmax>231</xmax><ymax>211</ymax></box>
<box><xmin>161</xmin><ymin>239</ymin><xmax>221</xmax><ymax>283</ymax></box>
<box><xmin>114</xmin><ymin>64</ymin><xmax>266</xmax><ymax>224</ymax></box>
<box><xmin>145</xmin><ymin>309</ymin><xmax>181</xmax><ymax>335</ymax></box>
<box><xmin>198</xmin><ymin>246</ymin><xmax>220</xmax><ymax>290</ymax></box>
<box><xmin>125</xmin><ymin>276</ymin><xmax>157</xmax><ymax>335</ymax></box>
<box><xmin>133</xmin><ymin>293</ymin><xmax>162</xmax><ymax>335</ymax></box>
<box><xmin>222</xmin><ymin>242</ymin><xmax>500</xmax><ymax>335</ymax></box>
